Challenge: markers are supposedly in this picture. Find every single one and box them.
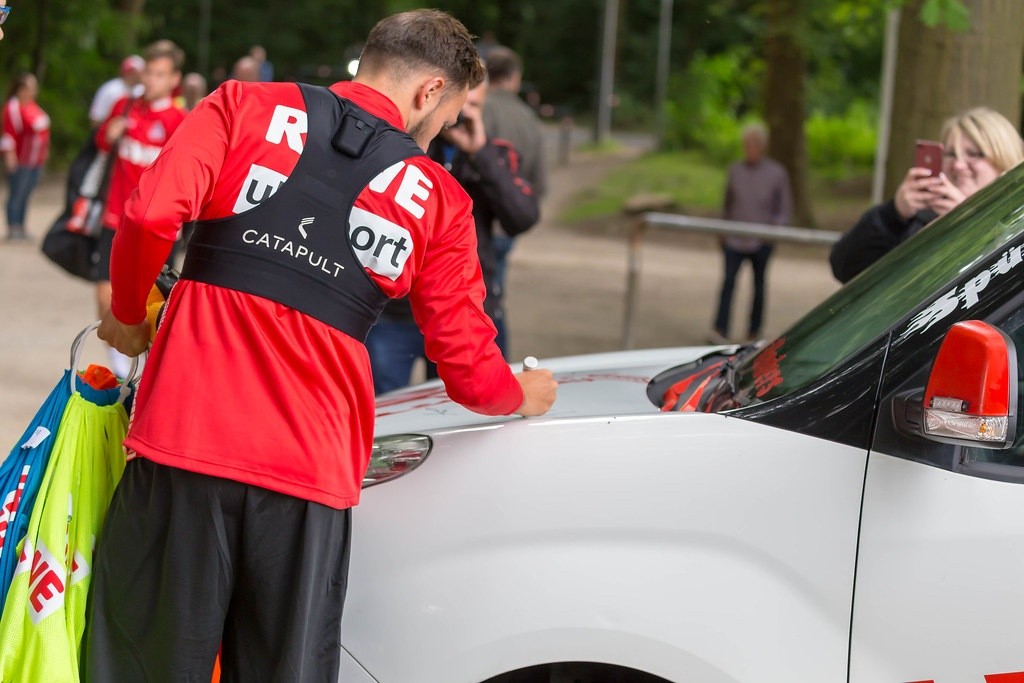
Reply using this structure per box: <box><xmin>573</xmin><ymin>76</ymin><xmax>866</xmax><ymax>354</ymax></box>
<box><xmin>522</xmin><ymin>355</ymin><xmax>539</xmax><ymax>419</ymax></box>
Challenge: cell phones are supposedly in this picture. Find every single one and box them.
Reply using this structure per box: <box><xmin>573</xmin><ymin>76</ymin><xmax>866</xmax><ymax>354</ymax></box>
<box><xmin>914</xmin><ymin>138</ymin><xmax>946</xmax><ymax>192</ymax></box>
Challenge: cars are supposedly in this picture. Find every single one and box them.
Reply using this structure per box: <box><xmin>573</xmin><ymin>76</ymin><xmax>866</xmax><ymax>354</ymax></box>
<box><xmin>339</xmin><ymin>160</ymin><xmax>1023</xmax><ymax>682</ymax></box>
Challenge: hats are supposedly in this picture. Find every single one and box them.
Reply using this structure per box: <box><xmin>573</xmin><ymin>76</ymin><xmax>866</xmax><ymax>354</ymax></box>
<box><xmin>120</xmin><ymin>53</ymin><xmax>143</xmax><ymax>71</ymax></box>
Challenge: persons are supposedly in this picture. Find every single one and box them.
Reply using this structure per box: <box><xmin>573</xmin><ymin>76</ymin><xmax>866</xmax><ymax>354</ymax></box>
<box><xmin>79</xmin><ymin>9</ymin><xmax>558</xmax><ymax>683</ymax></box>
<box><xmin>704</xmin><ymin>117</ymin><xmax>793</xmax><ymax>350</ymax></box>
<box><xmin>0</xmin><ymin>72</ymin><xmax>53</xmax><ymax>242</ymax></box>
<box><xmin>363</xmin><ymin>41</ymin><xmax>546</xmax><ymax>399</ymax></box>
<box><xmin>827</xmin><ymin>106</ymin><xmax>1024</xmax><ymax>285</ymax></box>
<box><xmin>68</xmin><ymin>41</ymin><xmax>275</xmax><ymax>319</ymax></box>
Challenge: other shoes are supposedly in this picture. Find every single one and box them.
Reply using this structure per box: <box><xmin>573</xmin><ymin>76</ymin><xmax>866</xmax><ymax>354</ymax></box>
<box><xmin>708</xmin><ymin>331</ymin><xmax>726</xmax><ymax>347</ymax></box>
<box><xmin>7</xmin><ymin>223</ymin><xmax>33</xmax><ymax>243</ymax></box>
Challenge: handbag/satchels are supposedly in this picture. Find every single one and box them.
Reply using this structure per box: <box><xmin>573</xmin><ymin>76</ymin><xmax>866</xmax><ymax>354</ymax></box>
<box><xmin>0</xmin><ymin>319</ymin><xmax>141</xmax><ymax>683</ymax></box>
<box><xmin>43</xmin><ymin>94</ymin><xmax>134</xmax><ymax>284</ymax></box>
